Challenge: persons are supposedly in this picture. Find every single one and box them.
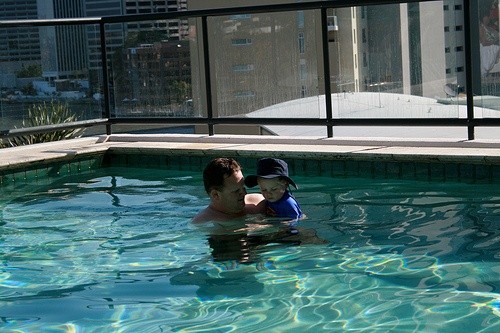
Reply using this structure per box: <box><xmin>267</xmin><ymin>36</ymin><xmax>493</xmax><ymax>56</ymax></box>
<box><xmin>257</xmin><ymin>157</ymin><xmax>302</xmax><ymax>223</ymax></box>
<box><xmin>191</xmin><ymin>158</ymin><xmax>265</xmax><ymax>224</ymax></box>
<box><xmin>480</xmin><ymin>15</ymin><xmax>500</xmax><ymax>46</ymax></box>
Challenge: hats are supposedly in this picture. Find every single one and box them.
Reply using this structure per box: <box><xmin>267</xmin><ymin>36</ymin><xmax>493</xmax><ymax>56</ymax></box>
<box><xmin>244</xmin><ymin>157</ymin><xmax>299</xmax><ymax>190</ymax></box>
